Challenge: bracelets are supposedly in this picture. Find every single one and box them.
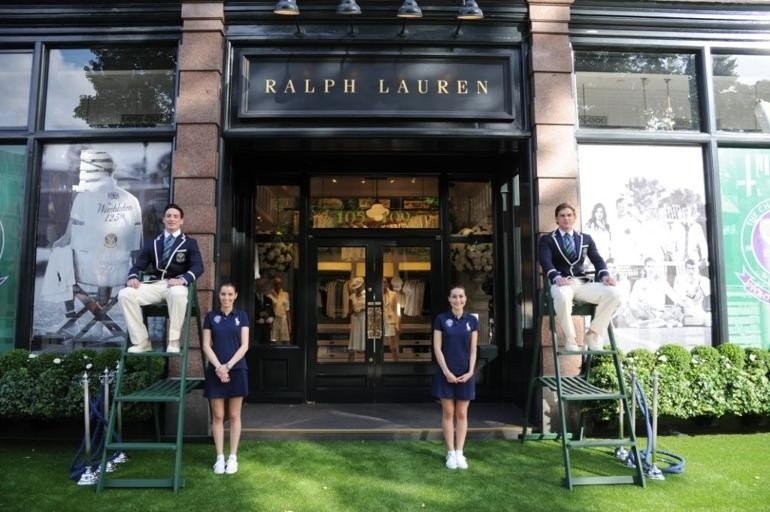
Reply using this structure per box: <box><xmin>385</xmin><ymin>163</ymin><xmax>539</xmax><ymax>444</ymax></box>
<box><xmin>225</xmin><ymin>363</ymin><xmax>231</xmax><ymax>371</ymax></box>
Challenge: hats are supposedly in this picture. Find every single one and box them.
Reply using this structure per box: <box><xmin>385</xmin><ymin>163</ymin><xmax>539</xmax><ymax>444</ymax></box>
<box><xmin>350</xmin><ymin>277</ymin><xmax>364</xmax><ymax>290</ymax></box>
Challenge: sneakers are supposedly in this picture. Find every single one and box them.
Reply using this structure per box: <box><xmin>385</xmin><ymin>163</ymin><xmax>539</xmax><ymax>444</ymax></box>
<box><xmin>445</xmin><ymin>454</ymin><xmax>468</xmax><ymax>469</ymax></box>
<box><xmin>583</xmin><ymin>331</ymin><xmax>604</xmax><ymax>352</ymax></box>
<box><xmin>565</xmin><ymin>338</ymin><xmax>578</xmax><ymax>352</ymax></box>
<box><xmin>213</xmin><ymin>459</ymin><xmax>238</xmax><ymax>474</ymax></box>
<box><xmin>128</xmin><ymin>341</ymin><xmax>152</xmax><ymax>353</ymax></box>
<box><xmin>166</xmin><ymin>342</ymin><xmax>181</xmax><ymax>353</ymax></box>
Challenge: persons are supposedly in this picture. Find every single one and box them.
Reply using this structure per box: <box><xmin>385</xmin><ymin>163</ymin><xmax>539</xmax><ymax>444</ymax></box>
<box><xmin>40</xmin><ymin>150</ymin><xmax>144</xmax><ymax>341</ymax></box>
<box><xmin>347</xmin><ymin>278</ymin><xmax>366</xmax><ymax>362</ymax></box>
<box><xmin>266</xmin><ymin>276</ymin><xmax>292</xmax><ymax>344</ymax></box>
<box><xmin>255</xmin><ymin>278</ymin><xmax>275</xmax><ymax>345</ymax></box>
<box><xmin>46</xmin><ymin>171</ymin><xmax>74</xmax><ymax>243</ymax></box>
<box><xmin>433</xmin><ymin>285</ymin><xmax>480</xmax><ymax>470</ymax></box>
<box><xmin>584</xmin><ymin>197</ymin><xmax>712</xmax><ymax>330</ymax></box>
<box><xmin>116</xmin><ymin>203</ymin><xmax>204</xmax><ymax>354</ymax></box>
<box><xmin>383</xmin><ymin>278</ymin><xmax>401</xmax><ymax>361</ymax></box>
<box><xmin>201</xmin><ymin>281</ymin><xmax>251</xmax><ymax>475</ymax></box>
<box><xmin>537</xmin><ymin>203</ymin><xmax>618</xmax><ymax>353</ymax></box>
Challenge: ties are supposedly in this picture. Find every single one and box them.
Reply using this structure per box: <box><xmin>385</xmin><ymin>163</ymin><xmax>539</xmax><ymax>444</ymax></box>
<box><xmin>160</xmin><ymin>234</ymin><xmax>172</xmax><ymax>269</ymax></box>
<box><xmin>565</xmin><ymin>233</ymin><xmax>576</xmax><ymax>263</ymax></box>
